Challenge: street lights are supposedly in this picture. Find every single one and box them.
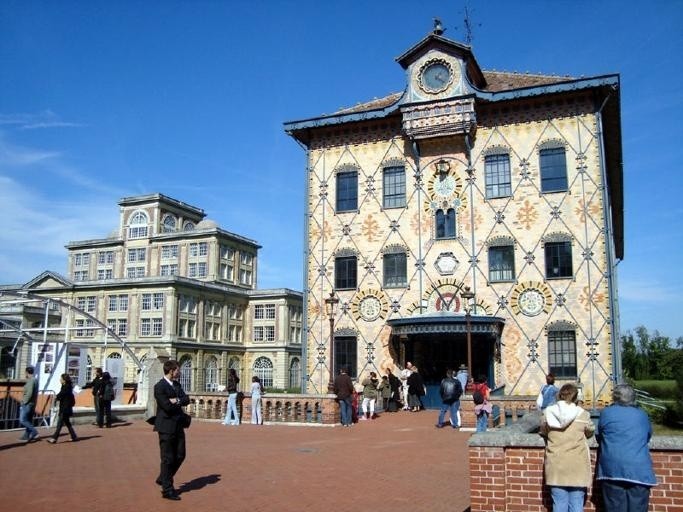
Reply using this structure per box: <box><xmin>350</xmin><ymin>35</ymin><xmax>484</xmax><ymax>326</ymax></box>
<box><xmin>325</xmin><ymin>289</ymin><xmax>339</xmax><ymax>393</ymax></box>
<box><xmin>460</xmin><ymin>284</ymin><xmax>474</xmax><ymax>394</ymax></box>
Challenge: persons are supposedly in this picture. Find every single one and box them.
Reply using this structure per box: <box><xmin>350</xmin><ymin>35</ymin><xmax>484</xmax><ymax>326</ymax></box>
<box><xmin>437</xmin><ymin>363</ymin><xmax>491</xmax><ymax>435</ymax></box>
<box><xmin>539</xmin><ymin>374</ymin><xmax>558</xmax><ymax>410</ymax></box>
<box><xmin>541</xmin><ymin>384</ymin><xmax>595</xmax><ymax>512</ymax></box>
<box><xmin>46</xmin><ymin>375</ymin><xmax>79</xmax><ymax>443</ymax></box>
<box><xmin>333</xmin><ymin>357</ymin><xmax>427</xmax><ymax>427</ymax></box>
<box><xmin>250</xmin><ymin>376</ymin><xmax>263</xmax><ymax>425</ymax></box>
<box><xmin>93</xmin><ymin>372</ymin><xmax>115</xmax><ymax>428</ymax></box>
<box><xmin>222</xmin><ymin>368</ymin><xmax>240</xmax><ymax>426</ymax></box>
<box><xmin>82</xmin><ymin>367</ymin><xmax>105</xmax><ymax>426</ymax></box>
<box><xmin>145</xmin><ymin>359</ymin><xmax>190</xmax><ymax>500</ymax></box>
<box><xmin>16</xmin><ymin>367</ymin><xmax>41</xmax><ymax>443</ymax></box>
<box><xmin>597</xmin><ymin>384</ymin><xmax>658</xmax><ymax>511</ymax></box>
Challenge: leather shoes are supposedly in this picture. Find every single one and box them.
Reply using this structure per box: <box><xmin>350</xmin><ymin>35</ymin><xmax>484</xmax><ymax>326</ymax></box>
<box><xmin>156</xmin><ymin>478</ymin><xmax>181</xmax><ymax>500</ymax></box>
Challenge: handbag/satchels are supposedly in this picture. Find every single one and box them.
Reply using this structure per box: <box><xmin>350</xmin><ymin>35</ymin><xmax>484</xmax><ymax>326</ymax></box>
<box><xmin>49</xmin><ymin>406</ymin><xmax>61</xmax><ymax>427</ymax></box>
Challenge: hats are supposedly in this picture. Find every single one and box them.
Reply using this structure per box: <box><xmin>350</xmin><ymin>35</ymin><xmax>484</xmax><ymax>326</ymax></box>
<box><xmin>458</xmin><ymin>364</ymin><xmax>469</xmax><ymax>370</ymax></box>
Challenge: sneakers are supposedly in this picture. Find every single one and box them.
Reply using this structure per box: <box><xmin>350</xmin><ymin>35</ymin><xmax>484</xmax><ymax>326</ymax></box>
<box><xmin>341</xmin><ymin>408</ymin><xmax>421</xmax><ymax>427</ymax></box>
<box><xmin>220</xmin><ymin>420</ymin><xmax>263</xmax><ymax>426</ymax></box>
<box><xmin>16</xmin><ymin>425</ymin><xmax>114</xmax><ymax>444</ymax></box>
<box><xmin>435</xmin><ymin>422</ymin><xmax>463</xmax><ymax>429</ymax></box>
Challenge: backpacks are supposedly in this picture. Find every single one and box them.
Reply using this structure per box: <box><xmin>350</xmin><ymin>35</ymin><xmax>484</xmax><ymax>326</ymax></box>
<box><xmin>104</xmin><ymin>382</ymin><xmax>115</xmax><ymax>401</ymax></box>
<box><xmin>536</xmin><ymin>384</ymin><xmax>553</xmax><ymax>408</ymax></box>
<box><xmin>473</xmin><ymin>384</ymin><xmax>486</xmax><ymax>404</ymax></box>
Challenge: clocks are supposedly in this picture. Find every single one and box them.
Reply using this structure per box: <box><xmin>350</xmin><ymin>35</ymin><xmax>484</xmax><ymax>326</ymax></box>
<box><xmin>416</xmin><ymin>57</ymin><xmax>454</xmax><ymax>94</ymax></box>
